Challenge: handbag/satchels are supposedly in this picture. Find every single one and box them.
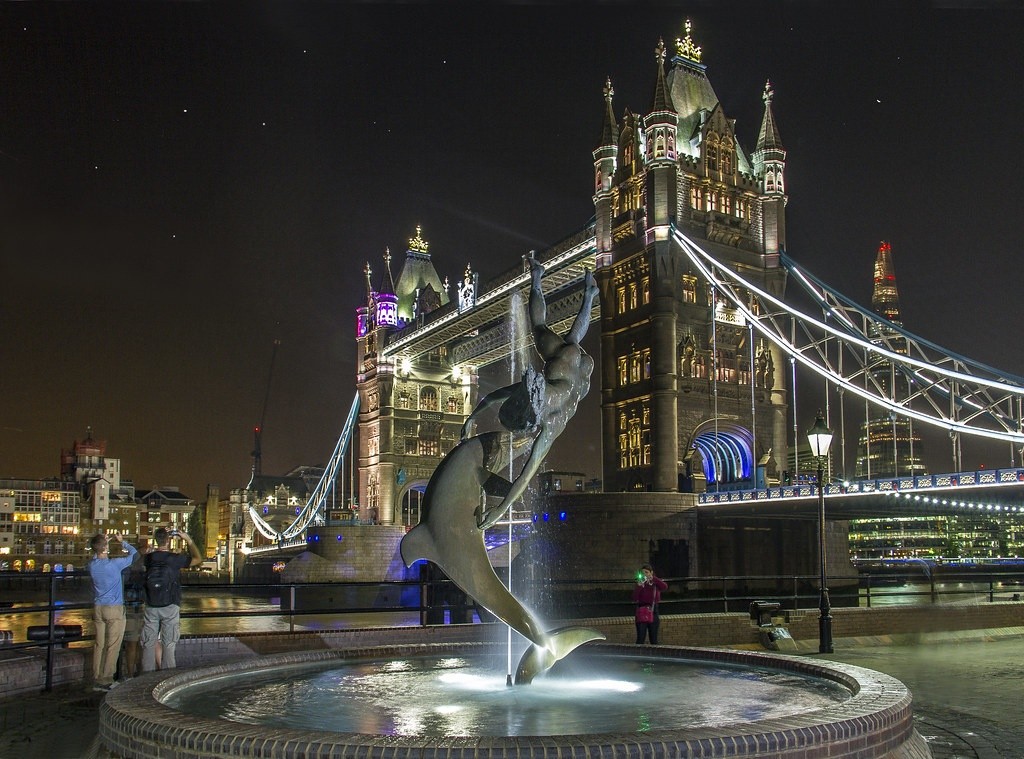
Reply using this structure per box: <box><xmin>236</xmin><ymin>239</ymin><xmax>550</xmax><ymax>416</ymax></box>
<box><xmin>635</xmin><ymin>605</ymin><xmax>654</xmax><ymax>624</ymax></box>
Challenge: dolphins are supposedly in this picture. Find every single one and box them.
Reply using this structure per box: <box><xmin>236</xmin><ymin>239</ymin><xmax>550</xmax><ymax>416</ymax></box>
<box><xmin>399</xmin><ymin>430</ymin><xmax>607</xmax><ymax>686</ymax></box>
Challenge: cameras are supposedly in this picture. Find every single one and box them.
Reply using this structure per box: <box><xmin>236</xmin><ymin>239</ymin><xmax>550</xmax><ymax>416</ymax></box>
<box><xmin>168</xmin><ymin>530</ymin><xmax>181</xmax><ymax>535</ymax></box>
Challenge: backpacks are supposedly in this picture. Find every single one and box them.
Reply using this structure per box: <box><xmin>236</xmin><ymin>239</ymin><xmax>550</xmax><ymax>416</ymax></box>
<box><xmin>144</xmin><ymin>552</ymin><xmax>180</xmax><ymax>608</ymax></box>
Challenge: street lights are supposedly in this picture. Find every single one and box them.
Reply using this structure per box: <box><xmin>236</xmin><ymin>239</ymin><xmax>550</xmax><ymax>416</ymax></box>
<box><xmin>808</xmin><ymin>407</ymin><xmax>834</xmax><ymax>655</ymax></box>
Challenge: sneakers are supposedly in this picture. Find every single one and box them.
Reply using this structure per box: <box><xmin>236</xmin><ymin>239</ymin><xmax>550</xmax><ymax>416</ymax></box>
<box><xmin>92</xmin><ymin>680</ymin><xmax>120</xmax><ymax>692</ymax></box>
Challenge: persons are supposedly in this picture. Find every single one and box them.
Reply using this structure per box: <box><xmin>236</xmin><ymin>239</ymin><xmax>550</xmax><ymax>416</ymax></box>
<box><xmin>461</xmin><ymin>255</ymin><xmax>599</xmax><ymax>531</ymax></box>
<box><xmin>460</xmin><ymin>278</ymin><xmax>473</xmax><ymax>310</ymax></box>
<box><xmin>89</xmin><ymin>527</ymin><xmax>203</xmax><ymax>693</ymax></box>
<box><xmin>630</xmin><ymin>564</ymin><xmax>668</xmax><ymax>645</ymax></box>
